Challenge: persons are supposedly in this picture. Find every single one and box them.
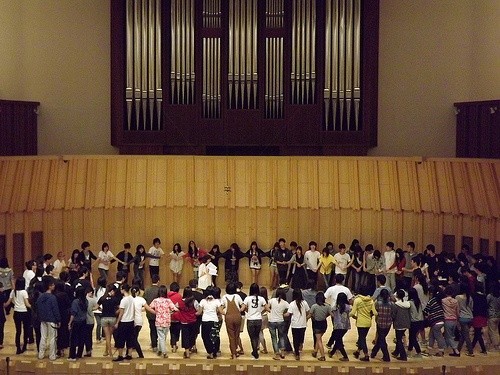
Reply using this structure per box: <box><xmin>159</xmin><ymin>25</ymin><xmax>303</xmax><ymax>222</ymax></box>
<box><xmin>0</xmin><ymin>237</ymin><xmax>500</xmax><ymax>362</ymax></box>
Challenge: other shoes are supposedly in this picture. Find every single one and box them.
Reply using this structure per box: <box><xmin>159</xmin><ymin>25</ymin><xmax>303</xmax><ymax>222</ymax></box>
<box><xmin>171</xmin><ymin>345</ymin><xmax>178</xmax><ymax>354</ymax></box>
<box><xmin>339</xmin><ymin>357</ymin><xmax>349</xmax><ymax>361</ymax></box>
<box><xmin>311</xmin><ymin>351</ymin><xmax>326</xmax><ymax>361</ymax></box>
<box><xmin>328</xmin><ymin>350</ymin><xmax>332</xmax><ymax>357</ymax></box>
<box><xmin>353</xmin><ymin>351</ymin><xmax>360</xmax><ymax>358</ymax></box>
<box><xmin>449</xmin><ymin>349</ymin><xmax>489</xmax><ymax>357</ymax></box>
<box><xmin>97</xmin><ymin>337</ymin><xmax>106</xmax><ymax>344</ymax></box>
<box><xmin>190</xmin><ymin>347</ymin><xmax>198</xmax><ymax>354</ymax></box>
<box><xmin>183</xmin><ymin>351</ymin><xmax>191</xmax><ymax>359</ymax></box>
<box><xmin>391</xmin><ymin>350</ymin><xmax>444</xmax><ymax>361</ymax></box>
<box><xmin>231</xmin><ymin>354</ymin><xmax>240</xmax><ymax>357</ymax></box>
<box><xmin>325</xmin><ymin>344</ymin><xmax>331</xmax><ymax>349</ymax></box>
<box><xmin>103</xmin><ymin>351</ymin><xmax>144</xmax><ymax>361</ymax></box>
<box><xmin>250</xmin><ymin>347</ymin><xmax>300</xmax><ymax>361</ymax></box>
<box><xmin>56</xmin><ymin>351</ymin><xmax>64</xmax><ymax>357</ymax></box>
<box><xmin>360</xmin><ymin>355</ymin><xmax>370</xmax><ymax>361</ymax></box>
<box><xmin>84</xmin><ymin>353</ymin><xmax>92</xmax><ymax>357</ymax></box>
<box><xmin>206</xmin><ymin>352</ymin><xmax>217</xmax><ymax>360</ymax></box>
<box><xmin>237</xmin><ymin>350</ymin><xmax>244</xmax><ymax>354</ymax></box>
<box><xmin>66</xmin><ymin>357</ymin><xmax>76</xmax><ymax>362</ymax></box>
<box><xmin>382</xmin><ymin>358</ymin><xmax>390</xmax><ymax>362</ymax></box>
<box><xmin>16</xmin><ymin>347</ymin><xmax>27</xmax><ymax>354</ymax></box>
<box><xmin>28</xmin><ymin>337</ymin><xmax>34</xmax><ymax>345</ymax></box>
<box><xmin>156</xmin><ymin>351</ymin><xmax>169</xmax><ymax>358</ymax></box>
<box><xmin>371</xmin><ymin>353</ymin><xmax>375</xmax><ymax>358</ymax></box>
